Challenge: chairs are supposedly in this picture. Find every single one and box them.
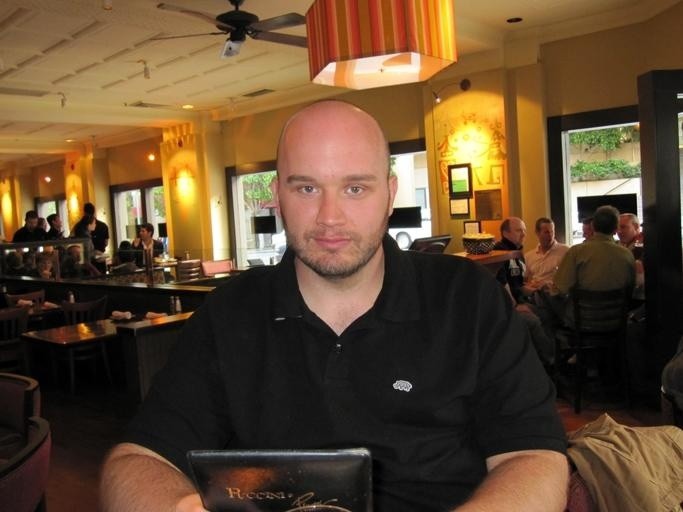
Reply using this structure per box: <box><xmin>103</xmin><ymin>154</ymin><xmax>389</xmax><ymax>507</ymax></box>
<box><xmin>566</xmin><ymin>283</ymin><xmax>636</xmax><ymax>415</ymax></box>
<box><xmin>172</xmin><ymin>256</ymin><xmax>203</xmax><ymax>284</ymax></box>
<box><xmin>201</xmin><ymin>256</ymin><xmax>238</xmax><ymax>278</ymax></box>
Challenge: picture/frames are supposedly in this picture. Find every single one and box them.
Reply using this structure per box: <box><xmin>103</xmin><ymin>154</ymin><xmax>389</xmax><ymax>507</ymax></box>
<box><xmin>449</xmin><ymin>196</ymin><xmax>469</xmax><ymax>215</ymax></box>
<box><xmin>462</xmin><ymin>220</ymin><xmax>481</xmax><ymax>236</ymax></box>
<box><xmin>447</xmin><ymin>164</ymin><xmax>473</xmax><ymax>199</ymax></box>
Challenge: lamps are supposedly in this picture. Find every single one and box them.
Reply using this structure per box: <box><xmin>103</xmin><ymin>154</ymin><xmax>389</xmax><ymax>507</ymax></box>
<box><xmin>305</xmin><ymin>2</ymin><xmax>456</xmax><ymax>91</ymax></box>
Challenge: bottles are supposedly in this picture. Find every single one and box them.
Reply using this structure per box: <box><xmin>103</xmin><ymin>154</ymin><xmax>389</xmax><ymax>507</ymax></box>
<box><xmin>174</xmin><ymin>296</ymin><xmax>181</xmax><ymax>312</ymax></box>
<box><xmin>166</xmin><ymin>296</ymin><xmax>175</xmax><ymax>315</ymax></box>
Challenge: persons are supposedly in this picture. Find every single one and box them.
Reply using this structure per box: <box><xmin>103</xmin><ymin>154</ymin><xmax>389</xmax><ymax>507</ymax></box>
<box><xmin>491</xmin><ymin>205</ymin><xmax>646</xmax><ymax>399</ymax></box>
<box><xmin>96</xmin><ymin>98</ymin><xmax>572</xmax><ymax>511</ymax></box>
<box><xmin>0</xmin><ymin>202</ymin><xmax>165</xmax><ymax>281</ymax></box>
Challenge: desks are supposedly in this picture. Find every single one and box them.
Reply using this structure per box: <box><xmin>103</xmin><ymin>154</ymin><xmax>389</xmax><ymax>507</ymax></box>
<box><xmin>152</xmin><ymin>259</ymin><xmax>179</xmax><ymax>269</ymax></box>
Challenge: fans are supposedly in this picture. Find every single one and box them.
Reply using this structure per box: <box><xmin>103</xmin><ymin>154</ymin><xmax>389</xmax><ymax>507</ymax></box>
<box><xmin>150</xmin><ymin>0</ymin><xmax>312</xmax><ymax>65</ymax></box>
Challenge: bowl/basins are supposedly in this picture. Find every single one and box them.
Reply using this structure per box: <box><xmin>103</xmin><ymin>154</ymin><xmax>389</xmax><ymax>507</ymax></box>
<box><xmin>462</xmin><ymin>234</ymin><xmax>495</xmax><ymax>254</ymax></box>
